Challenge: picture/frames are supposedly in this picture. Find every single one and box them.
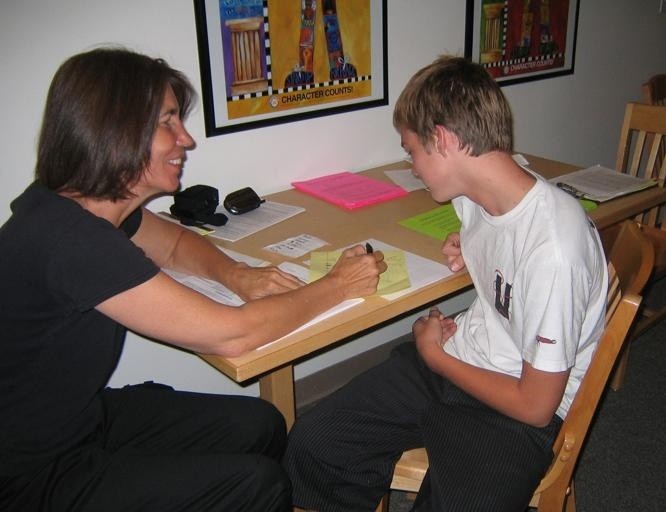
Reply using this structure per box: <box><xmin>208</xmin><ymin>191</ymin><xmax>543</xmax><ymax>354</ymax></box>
<box><xmin>193</xmin><ymin>0</ymin><xmax>389</xmax><ymax>138</ymax></box>
<box><xmin>464</xmin><ymin>0</ymin><xmax>580</xmax><ymax>87</ymax></box>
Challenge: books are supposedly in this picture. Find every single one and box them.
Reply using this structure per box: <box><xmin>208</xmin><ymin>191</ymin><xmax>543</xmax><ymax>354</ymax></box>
<box><xmin>547</xmin><ymin>164</ymin><xmax>656</xmax><ymax>204</ymax></box>
<box><xmin>160</xmin><ymin>244</ymin><xmax>365</xmax><ymax>352</ymax></box>
<box><xmin>290</xmin><ymin>171</ymin><xmax>408</xmax><ymax>211</ymax></box>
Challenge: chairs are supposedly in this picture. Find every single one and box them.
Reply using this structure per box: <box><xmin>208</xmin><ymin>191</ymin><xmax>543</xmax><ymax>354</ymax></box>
<box><xmin>641</xmin><ymin>74</ymin><xmax>666</xmax><ymax>178</ymax></box>
<box><xmin>375</xmin><ymin>218</ymin><xmax>656</xmax><ymax>512</ymax></box>
<box><xmin>608</xmin><ymin>102</ymin><xmax>666</xmax><ymax>391</ymax></box>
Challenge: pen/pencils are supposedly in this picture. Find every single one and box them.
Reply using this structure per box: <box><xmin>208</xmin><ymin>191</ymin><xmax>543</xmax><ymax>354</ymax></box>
<box><xmin>366</xmin><ymin>243</ymin><xmax>373</xmax><ymax>254</ymax></box>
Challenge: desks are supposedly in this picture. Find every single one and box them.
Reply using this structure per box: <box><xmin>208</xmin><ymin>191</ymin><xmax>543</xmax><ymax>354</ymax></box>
<box><xmin>156</xmin><ymin>150</ymin><xmax>665</xmax><ymax>435</ymax></box>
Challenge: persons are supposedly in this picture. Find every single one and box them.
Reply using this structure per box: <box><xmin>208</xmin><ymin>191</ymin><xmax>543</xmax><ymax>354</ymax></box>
<box><xmin>288</xmin><ymin>52</ymin><xmax>610</xmax><ymax>510</ymax></box>
<box><xmin>0</xmin><ymin>46</ymin><xmax>388</xmax><ymax>512</ymax></box>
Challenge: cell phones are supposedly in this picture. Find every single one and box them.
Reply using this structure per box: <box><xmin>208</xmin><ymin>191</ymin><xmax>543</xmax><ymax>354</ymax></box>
<box><xmin>224</xmin><ymin>187</ymin><xmax>265</xmax><ymax>215</ymax></box>
